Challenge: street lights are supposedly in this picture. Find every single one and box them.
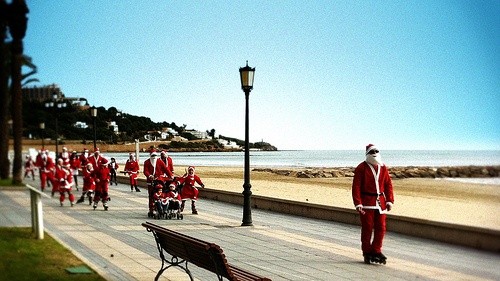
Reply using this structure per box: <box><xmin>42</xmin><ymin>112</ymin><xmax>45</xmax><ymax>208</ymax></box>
<box><xmin>239</xmin><ymin>59</ymin><xmax>256</xmax><ymax>226</ymax></box>
<box><xmin>45</xmin><ymin>94</ymin><xmax>67</xmax><ymax>166</ymax></box>
<box><xmin>90</xmin><ymin>105</ymin><xmax>98</xmax><ymax>150</ymax></box>
<box><xmin>40</xmin><ymin>121</ymin><xmax>46</xmax><ymax>150</ymax></box>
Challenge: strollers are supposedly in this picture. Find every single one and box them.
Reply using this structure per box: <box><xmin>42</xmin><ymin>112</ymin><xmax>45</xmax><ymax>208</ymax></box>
<box><xmin>151</xmin><ymin>177</ymin><xmax>184</xmax><ymax>220</ymax></box>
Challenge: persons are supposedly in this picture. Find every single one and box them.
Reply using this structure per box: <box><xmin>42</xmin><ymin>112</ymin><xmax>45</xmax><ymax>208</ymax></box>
<box><xmin>107</xmin><ymin>157</ymin><xmax>119</xmax><ymax>186</ymax></box>
<box><xmin>124</xmin><ymin>152</ymin><xmax>141</xmax><ymax>192</ymax></box>
<box><xmin>23</xmin><ymin>146</ymin><xmax>119</xmax><ymax>208</ymax></box>
<box><xmin>352</xmin><ymin>144</ymin><xmax>394</xmax><ymax>260</ymax></box>
<box><xmin>143</xmin><ymin>147</ymin><xmax>205</xmax><ymax>215</ymax></box>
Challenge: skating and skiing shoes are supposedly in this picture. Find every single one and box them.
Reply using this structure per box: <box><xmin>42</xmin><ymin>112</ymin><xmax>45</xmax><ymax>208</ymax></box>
<box><xmin>370</xmin><ymin>250</ymin><xmax>387</xmax><ymax>264</ymax></box>
<box><xmin>103</xmin><ymin>202</ymin><xmax>109</xmax><ymax>210</ymax></box>
<box><xmin>89</xmin><ymin>197</ymin><xmax>93</xmax><ymax>205</ymax></box>
<box><xmin>131</xmin><ymin>187</ymin><xmax>134</xmax><ymax>192</ymax></box>
<box><xmin>60</xmin><ymin>202</ymin><xmax>64</xmax><ymax>206</ymax></box>
<box><xmin>135</xmin><ymin>187</ymin><xmax>141</xmax><ymax>192</ymax></box>
<box><xmin>93</xmin><ymin>202</ymin><xmax>98</xmax><ymax>210</ymax></box>
<box><xmin>180</xmin><ymin>204</ymin><xmax>185</xmax><ymax>211</ymax></box>
<box><xmin>71</xmin><ymin>201</ymin><xmax>75</xmax><ymax>207</ymax></box>
<box><xmin>363</xmin><ymin>251</ymin><xmax>371</xmax><ymax>264</ymax></box>
<box><xmin>76</xmin><ymin>196</ymin><xmax>85</xmax><ymax>204</ymax></box>
<box><xmin>192</xmin><ymin>205</ymin><xmax>198</xmax><ymax>214</ymax></box>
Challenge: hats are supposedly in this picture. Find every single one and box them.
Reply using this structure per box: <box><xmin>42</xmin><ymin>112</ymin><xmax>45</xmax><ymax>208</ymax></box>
<box><xmin>111</xmin><ymin>157</ymin><xmax>115</xmax><ymax>160</ymax></box>
<box><xmin>150</xmin><ymin>148</ymin><xmax>157</xmax><ymax>157</ymax></box>
<box><xmin>129</xmin><ymin>152</ymin><xmax>135</xmax><ymax>157</ymax></box>
<box><xmin>365</xmin><ymin>144</ymin><xmax>378</xmax><ymax>155</ymax></box>
<box><xmin>188</xmin><ymin>165</ymin><xmax>196</xmax><ymax>171</ymax></box>
<box><xmin>169</xmin><ymin>182</ymin><xmax>177</xmax><ymax>188</ymax></box>
<box><xmin>160</xmin><ymin>149</ymin><xmax>167</xmax><ymax>155</ymax></box>
<box><xmin>156</xmin><ymin>183</ymin><xmax>164</xmax><ymax>189</ymax></box>
<box><xmin>24</xmin><ymin>146</ymin><xmax>109</xmax><ymax>168</ymax></box>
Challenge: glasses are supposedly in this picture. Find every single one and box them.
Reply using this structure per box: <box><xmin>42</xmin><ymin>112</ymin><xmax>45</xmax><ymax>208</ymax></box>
<box><xmin>369</xmin><ymin>150</ymin><xmax>379</xmax><ymax>154</ymax></box>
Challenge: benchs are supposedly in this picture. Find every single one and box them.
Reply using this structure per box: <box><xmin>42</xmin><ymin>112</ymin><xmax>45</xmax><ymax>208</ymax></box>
<box><xmin>141</xmin><ymin>221</ymin><xmax>272</xmax><ymax>281</ymax></box>
